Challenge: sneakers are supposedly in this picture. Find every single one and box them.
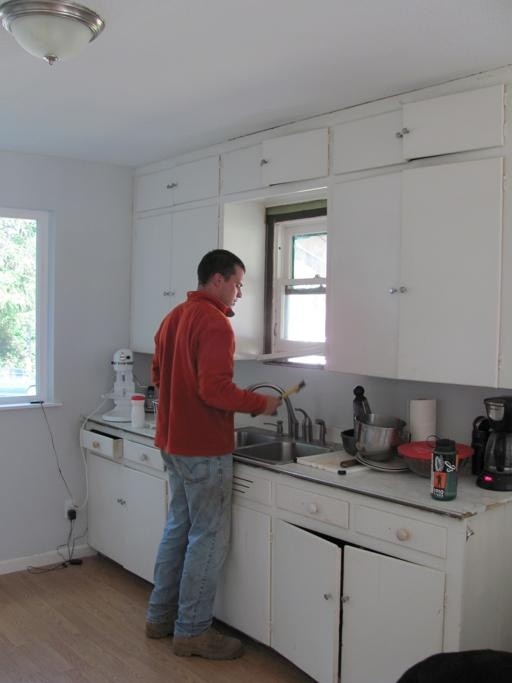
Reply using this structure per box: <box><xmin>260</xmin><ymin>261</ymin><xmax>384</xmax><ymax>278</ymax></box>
<box><xmin>171</xmin><ymin>625</ymin><xmax>245</xmax><ymax>661</ymax></box>
<box><xmin>144</xmin><ymin>612</ymin><xmax>179</xmax><ymax>639</ymax></box>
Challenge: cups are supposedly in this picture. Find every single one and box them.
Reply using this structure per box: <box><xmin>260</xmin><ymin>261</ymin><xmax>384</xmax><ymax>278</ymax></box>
<box><xmin>355</xmin><ymin>411</ymin><xmax>406</xmax><ymax>461</ymax></box>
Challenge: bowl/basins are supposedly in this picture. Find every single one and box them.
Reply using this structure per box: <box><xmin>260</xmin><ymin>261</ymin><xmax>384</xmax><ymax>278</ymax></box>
<box><xmin>340</xmin><ymin>429</ymin><xmax>356</xmax><ymax>457</ymax></box>
<box><xmin>397</xmin><ymin>441</ymin><xmax>474</xmax><ymax>477</ymax></box>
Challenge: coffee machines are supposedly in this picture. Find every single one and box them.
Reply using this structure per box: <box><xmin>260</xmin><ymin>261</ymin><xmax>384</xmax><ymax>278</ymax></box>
<box><xmin>476</xmin><ymin>396</ymin><xmax>512</xmax><ymax>489</ymax></box>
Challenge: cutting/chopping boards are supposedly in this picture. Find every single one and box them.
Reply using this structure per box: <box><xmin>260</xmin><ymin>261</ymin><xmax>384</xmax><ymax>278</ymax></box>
<box><xmin>296</xmin><ymin>448</ymin><xmax>369</xmax><ymax>474</ymax></box>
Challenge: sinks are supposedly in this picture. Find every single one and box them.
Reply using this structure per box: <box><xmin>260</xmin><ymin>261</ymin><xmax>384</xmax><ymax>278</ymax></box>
<box><xmin>233</xmin><ymin>439</ymin><xmax>338</xmax><ymax>464</ymax></box>
<box><xmin>233</xmin><ymin>426</ymin><xmax>282</xmax><ymax>448</ymax></box>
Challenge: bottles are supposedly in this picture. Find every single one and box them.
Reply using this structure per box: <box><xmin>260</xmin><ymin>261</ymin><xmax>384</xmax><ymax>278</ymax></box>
<box><xmin>131</xmin><ymin>394</ymin><xmax>145</xmax><ymax>427</ymax></box>
<box><xmin>470</xmin><ymin>415</ymin><xmax>488</xmax><ymax>473</ymax></box>
<box><xmin>426</xmin><ymin>434</ymin><xmax>463</xmax><ymax>502</ymax></box>
<box><xmin>139</xmin><ymin>386</ymin><xmax>159</xmax><ymax>429</ymax></box>
<box><xmin>351</xmin><ymin>385</ymin><xmax>372</xmax><ymax>422</ymax></box>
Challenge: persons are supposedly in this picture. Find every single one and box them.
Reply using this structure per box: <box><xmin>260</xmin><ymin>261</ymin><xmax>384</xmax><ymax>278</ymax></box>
<box><xmin>145</xmin><ymin>249</ymin><xmax>288</xmax><ymax>660</ymax></box>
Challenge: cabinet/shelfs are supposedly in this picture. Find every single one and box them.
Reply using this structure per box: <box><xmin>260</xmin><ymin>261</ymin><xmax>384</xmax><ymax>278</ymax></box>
<box><xmin>167</xmin><ymin>491</ymin><xmax>271</xmax><ymax>655</ymax></box>
<box><xmin>77</xmin><ymin>419</ymin><xmax>168</xmax><ymax>598</ymax></box>
<box><xmin>219</xmin><ymin>119</ymin><xmax>328</xmax><ymax>198</ymax></box>
<box><xmin>327</xmin><ymin>154</ymin><xmax>511</xmax><ymax>397</ymax></box>
<box><xmin>130</xmin><ymin>204</ymin><xmax>268</xmax><ymax>361</ymax></box>
<box><xmin>131</xmin><ymin>150</ymin><xmax>220</xmax><ymax>214</ymax></box>
<box><xmin>329</xmin><ymin>82</ymin><xmax>505</xmax><ymax>171</ymax></box>
<box><xmin>271</xmin><ymin>473</ymin><xmax>445</xmax><ymax>683</ymax></box>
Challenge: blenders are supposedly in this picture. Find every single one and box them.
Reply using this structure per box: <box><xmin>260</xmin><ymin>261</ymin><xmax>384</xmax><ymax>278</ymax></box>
<box><xmin>102</xmin><ymin>348</ymin><xmax>139</xmax><ymax>423</ymax></box>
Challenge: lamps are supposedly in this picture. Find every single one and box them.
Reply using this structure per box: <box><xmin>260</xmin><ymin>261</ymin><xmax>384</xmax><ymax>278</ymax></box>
<box><xmin>1</xmin><ymin>1</ymin><xmax>112</xmax><ymax>70</ymax></box>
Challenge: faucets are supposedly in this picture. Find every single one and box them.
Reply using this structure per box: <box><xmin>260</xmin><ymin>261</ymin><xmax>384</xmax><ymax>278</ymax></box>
<box><xmin>242</xmin><ymin>382</ymin><xmax>298</xmax><ymax>438</ymax></box>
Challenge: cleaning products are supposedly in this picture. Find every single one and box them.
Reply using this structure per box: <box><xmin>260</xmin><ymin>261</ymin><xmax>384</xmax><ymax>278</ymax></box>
<box><xmin>146</xmin><ymin>386</ymin><xmax>154</xmax><ymax>412</ymax></box>
<box><xmin>432</xmin><ymin>439</ymin><xmax>458</xmax><ymax>500</ymax></box>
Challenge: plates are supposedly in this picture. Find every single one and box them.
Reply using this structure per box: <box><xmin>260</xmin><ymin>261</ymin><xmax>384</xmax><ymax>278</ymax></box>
<box><xmin>355</xmin><ymin>451</ymin><xmax>409</xmax><ymax>474</ymax></box>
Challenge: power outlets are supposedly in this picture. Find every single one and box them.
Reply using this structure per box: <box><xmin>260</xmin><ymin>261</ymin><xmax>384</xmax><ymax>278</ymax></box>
<box><xmin>64</xmin><ymin>498</ymin><xmax>79</xmax><ymax>520</ymax></box>
